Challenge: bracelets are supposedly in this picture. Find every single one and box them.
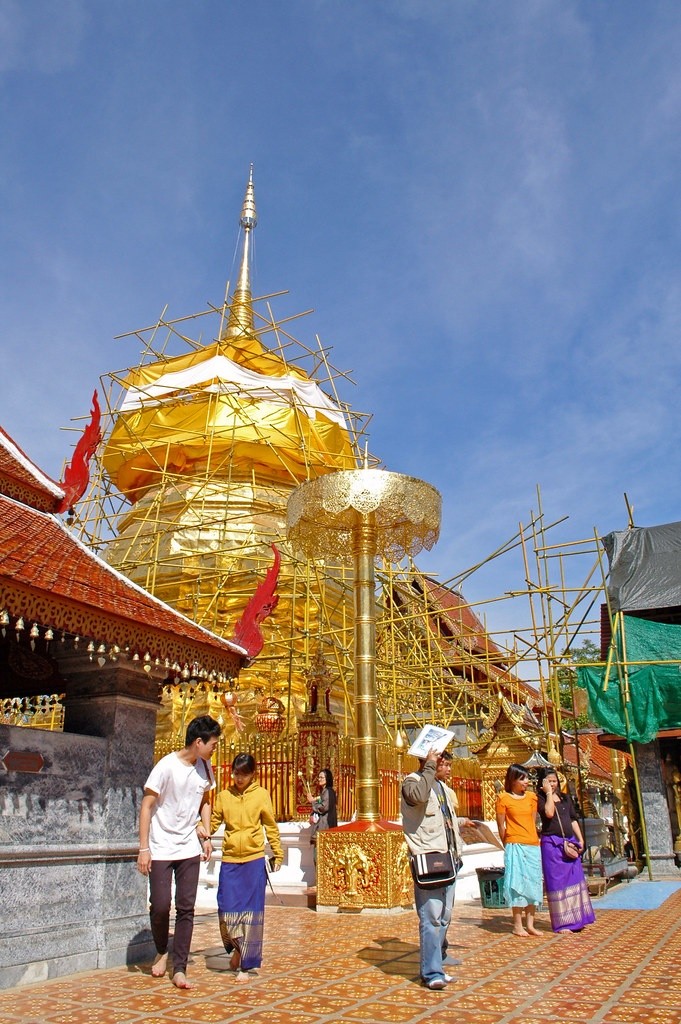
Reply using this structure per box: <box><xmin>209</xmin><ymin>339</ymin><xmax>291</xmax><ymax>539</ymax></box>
<box><xmin>138</xmin><ymin>848</ymin><xmax>150</xmax><ymax>852</ymax></box>
<box><xmin>546</xmin><ymin>792</ymin><xmax>552</xmax><ymax>795</ymax></box>
<box><xmin>204</xmin><ymin>836</ymin><xmax>212</xmax><ymax>840</ymax></box>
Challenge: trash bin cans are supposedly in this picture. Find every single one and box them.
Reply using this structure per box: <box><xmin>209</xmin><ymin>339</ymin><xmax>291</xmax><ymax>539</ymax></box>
<box><xmin>475</xmin><ymin>866</ymin><xmax>511</xmax><ymax>909</ymax></box>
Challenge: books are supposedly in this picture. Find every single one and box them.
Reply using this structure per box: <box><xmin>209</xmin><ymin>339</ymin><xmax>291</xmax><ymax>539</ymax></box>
<box><xmin>458</xmin><ymin>820</ymin><xmax>505</xmax><ymax>852</ymax></box>
<box><xmin>406</xmin><ymin>724</ymin><xmax>456</xmax><ymax>762</ymax></box>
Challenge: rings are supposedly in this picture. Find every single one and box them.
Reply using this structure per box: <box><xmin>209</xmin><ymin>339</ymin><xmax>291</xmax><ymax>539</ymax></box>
<box><xmin>431</xmin><ymin>752</ymin><xmax>434</xmax><ymax>754</ymax></box>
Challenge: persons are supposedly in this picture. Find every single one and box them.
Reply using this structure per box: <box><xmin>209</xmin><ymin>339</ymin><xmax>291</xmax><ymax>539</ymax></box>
<box><xmin>196</xmin><ymin>752</ymin><xmax>285</xmax><ymax>982</ymax></box>
<box><xmin>495</xmin><ymin>763</ymin><xmax>544</xmax><ymax>937</ymax></box>
<box><xmin>136</xmin><ymin>713</ymin><xmax>222</xmax><ymax>989</ymax></box>
<box><xmin>401</xmin><ymin>724</ymin><xmax>481</xmax><ymax>991</ymax></box>
<box><xmin>306</xmin><ymin>768</ymin><xmax>338</xmax><ymax>887</ymax></box>
<box><xmin>537</xmin><ymin>766</ymin><xmax>596</xmax><ymax>934</ymax></box>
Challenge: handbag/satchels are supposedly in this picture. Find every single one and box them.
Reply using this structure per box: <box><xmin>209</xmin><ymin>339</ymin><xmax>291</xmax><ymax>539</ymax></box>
<box><xmin>409</xmin><ymin>850</ymin><xmax>456</xmax><ymax>890</ymax></box>
<box><xmin>564</xmin><ymin>840</ymin><xmax>578</xmax><ymax>860</ymax></box>
